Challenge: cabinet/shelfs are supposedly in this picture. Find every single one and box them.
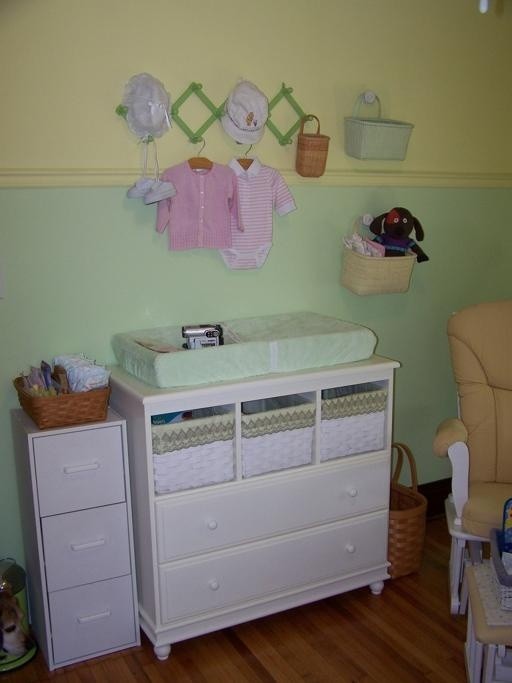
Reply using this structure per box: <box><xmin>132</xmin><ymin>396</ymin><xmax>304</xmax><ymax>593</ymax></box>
<box><xmin>108</xmin><ymin>355</ymin><xmax>400</xmax><ymax>662</ymax></box>
<box><xmin>11</xmin><ymin>404</ymin><xmax>142</xmax><ymax>672</ymax></box>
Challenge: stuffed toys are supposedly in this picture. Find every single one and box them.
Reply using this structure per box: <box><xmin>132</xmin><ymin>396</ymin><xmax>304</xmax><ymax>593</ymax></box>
<box><xmin>369</xmin><ymin>206</ymin><xmax>433</xmax><ymax>265</ymax></box>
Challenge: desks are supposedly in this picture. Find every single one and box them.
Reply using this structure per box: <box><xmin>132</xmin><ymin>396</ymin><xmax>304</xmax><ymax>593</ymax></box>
<box><xmin>464</xmin><ymin>565</ymin><xmax>512</xmax><ymax>683</ymax></box>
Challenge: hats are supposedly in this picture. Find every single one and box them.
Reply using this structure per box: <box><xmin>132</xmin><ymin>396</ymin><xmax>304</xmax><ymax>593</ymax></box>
<box><xmin>221</xmin><ymin>81</ymin><xmax>269</xmax><ymax>144</ymax></box>
<box><xmin>124</xmin><ymin>75</ymin><xmax>172</xmax><ymax>138</ymax></box>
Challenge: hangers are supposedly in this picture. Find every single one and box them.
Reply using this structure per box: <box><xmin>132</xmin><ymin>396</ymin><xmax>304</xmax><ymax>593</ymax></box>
<box><xmin>236</xmin><ymin>145</ymin><xmax>254</xmax><ymax>169</ymax></box>
<box><xmin>187</xmin><ymin>135</ymin><xmax>213</xmax><ymax>170</ymax></box>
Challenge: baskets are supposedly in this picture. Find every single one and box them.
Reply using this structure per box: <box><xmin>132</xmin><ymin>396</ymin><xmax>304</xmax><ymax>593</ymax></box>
<box><xmin>487</xmin><ymin>529</ymin><xmax>512</xmax><ymax>611</ymax></box>
<box><xmin>240</xmin><ymin>393</ymin><xmax>315</xmax><ymax>479</ymax></box>
<box><xmin>319</xmin><ymin>384</ymin><xmax>386</xmax><ymax>463</ymax></box>
<box><xmin>340</xmin><ymin>244</ymin><xmax>417</xmax><ymax>296</ymax></box>
<box><xmin>345</xmin><ymin>117</ymin><xmax>413</xmax><ymax>163</ymax></box>
<box><xmin>388</xmin><ymin>442</ymin><xmax>428</xmax><ymax>579</ymax></box>
<box><xmin>150</xmin><ymin>407</ymin><xmax>236</xmax><ymax>495</ymax></box>
<box><xmin>14</xmin><ymin>371</ymin><xmax>109</xmax><ymax>431</ymax></box>
<box><xmin>296</xmin><ymin>113</ymin><xmax>329</xmax><ymax>177</ymax></box>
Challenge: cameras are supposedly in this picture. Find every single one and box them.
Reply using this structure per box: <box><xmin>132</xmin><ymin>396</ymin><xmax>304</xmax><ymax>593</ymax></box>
<box><xmin>181</xmin><ymin>323</ymin><xmax>224</xmax><ymax>350</ymax></box>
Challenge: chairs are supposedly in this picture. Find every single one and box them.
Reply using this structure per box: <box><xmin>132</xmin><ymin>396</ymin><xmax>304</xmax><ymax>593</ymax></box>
<box><xmin>432</xmin><ymin>300</ymin><xmax>512</xmax><ymax>615</ymax></box>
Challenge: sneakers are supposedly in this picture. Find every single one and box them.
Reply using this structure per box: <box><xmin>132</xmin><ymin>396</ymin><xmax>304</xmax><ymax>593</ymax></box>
<box><xmin>127</xmin><ymin>177</ymin><xmax>175</xmax><ymax>204</ymax></box>
